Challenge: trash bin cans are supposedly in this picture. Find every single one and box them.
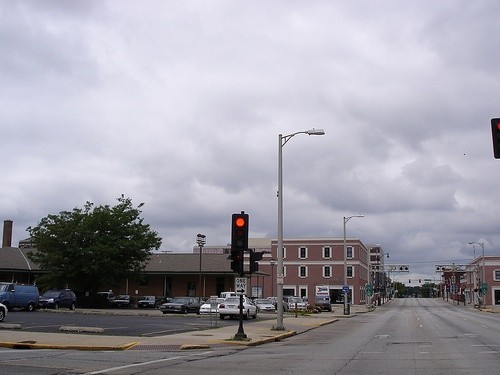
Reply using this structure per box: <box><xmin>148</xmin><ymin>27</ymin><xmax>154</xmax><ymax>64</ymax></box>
<box><xmin>344</xmin><ymin>303</ymin><xmax>350</xmax><ymax>315</ymax></box>
<box><xmin>389</xmin><ymin>294</ymin><xmax>391</xmax><ymax>300</ymax></box>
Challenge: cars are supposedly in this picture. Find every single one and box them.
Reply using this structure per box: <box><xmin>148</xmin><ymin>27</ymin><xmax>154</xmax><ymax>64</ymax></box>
<box><xmin>315</xmin><ymin>295</ymin><xmax>332</xmax><ymax>312</ymax></box>
<box><xmin>138</xmin><ymin>295</ymin><xmax>160</xmax><ymax>310</ymax></box>
<box><xmin>199</xmin><ymin>296</ymin><xmax>226</xmax><ymax>315</ymax></box>
<box><xmin>0</xmin><ymin>303</ymin><xmax>8</xmax><ymax>321</ymax></box>
<box><xmin>253</xmin><ymin>298</ymin><xmax>276</xmax><ymax>312</ymax></box>
<box><xmin>159</xmin><ymin>296</ymin><xmax>201</xmax><ymax>315</ymax></box>
<box><xmin>284</xmin><ymin>296</ymin><xmax>307</xmax><ymax>312</ymax></box>
<box><xmin>113</xmin><ymin>295</ymin><xmax>134</xmax><ymax>308</ymax></box>
<box><xmin>268</xmin><ymin>297</ymin><xmax>285</xmax><ymax>309</ymax></box>
<box><xmin>218</xmin><ymin>296</ymin><xmax>257</xmax><ymax>320</ymax></box>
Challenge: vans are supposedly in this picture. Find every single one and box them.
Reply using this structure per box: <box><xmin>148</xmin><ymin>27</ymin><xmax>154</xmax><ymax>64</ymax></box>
<box><xmin>97</xmin><ymin>292</ymin><xmax>115</xmax><ymax>304</ymax></box>
<box><xmin>221</xmin><ymin>292</ymin><xmax>246</xmax><ymax>299</ymax></box>
<box><xmin>0</xmin><ymin>282</ymin><xmax>40</xmax><ymax>312</ymax></box>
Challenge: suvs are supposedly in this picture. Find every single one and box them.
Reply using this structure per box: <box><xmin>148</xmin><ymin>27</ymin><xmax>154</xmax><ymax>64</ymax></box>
<box><xmin>39</xmin><ymin>289</ymin><xmax>77</xmax><ymax>311</ymax></box>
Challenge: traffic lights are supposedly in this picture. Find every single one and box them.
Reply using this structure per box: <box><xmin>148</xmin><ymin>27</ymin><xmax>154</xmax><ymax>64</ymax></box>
<box><xmin>490</xmin><ymin>117</ymin><xmax>500</xmax><ymax>158</ymax></box>
<box><xmin>230</xmin><ymin>250</ymin><xmax>242</xmax><ymax>273</ymax></box>
<box><xmin>230</xmin><ymin>213</ymin><xmax>249</xmax><ymax>251</ymax></box>
<box><xmin>249</xmin><ymin>249</ymin><xmax>264</xmax><ymax>275</ymax></box>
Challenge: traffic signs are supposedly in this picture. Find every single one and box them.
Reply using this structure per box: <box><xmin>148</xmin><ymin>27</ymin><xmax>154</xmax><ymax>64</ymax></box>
<box><xmin>234</xmin><ymin>278</ymin><xmax>247</xmax><ymax>296</ymax></box>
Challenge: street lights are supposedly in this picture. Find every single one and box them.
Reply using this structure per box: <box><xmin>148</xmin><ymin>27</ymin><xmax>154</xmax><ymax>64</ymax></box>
<box><xmin>195</xmin><ymin>233</ymin><xmax>206</xmax><ymax>302</ymax></box>
<box><xmin>343</xmin><ymin>215</ymin><xmax>365</xmax><ymax>316</ymax></box>
<box><xmin>275</xmin><ymin>127</ymin><xmax>326</xmax><ymax>330</ymax></box>
<box><xmin>468</xmin><ymin>241</ymin><xmax>487</xmax><ymax>308</ymax></box>
